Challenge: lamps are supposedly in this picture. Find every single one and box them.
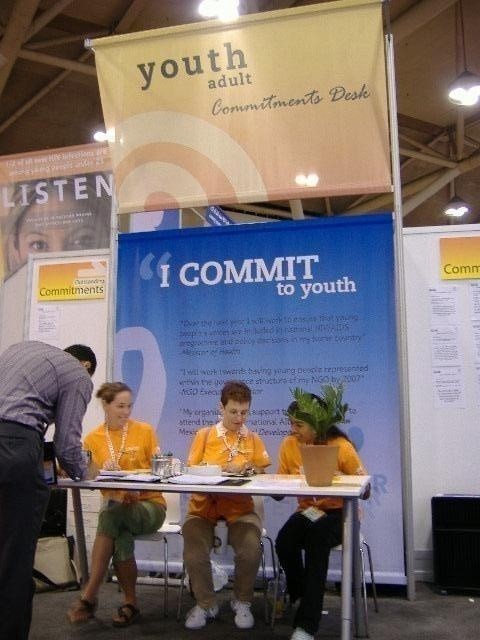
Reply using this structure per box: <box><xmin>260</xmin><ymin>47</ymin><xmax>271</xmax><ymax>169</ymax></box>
<box><xmin>444</xmin><ymin>164</ymin><xmax>470</xmax><ymax>221</ymax></box>
<box><xmin>447</xmin><ymin>2</ymin><xmax>480</xmax><ymax>107</ymax></box>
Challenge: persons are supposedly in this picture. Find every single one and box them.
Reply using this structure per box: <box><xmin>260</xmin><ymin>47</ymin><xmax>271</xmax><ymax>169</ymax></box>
<box><xmin>65</xmin><ymin>382</ymin><xmax>167</xmax><ymax>628</ymax></box>
<box><xmin>183</xmin><ymin>379</ymin><xmax>272</xmax><ymax>630</ymax></box>
<box><xmin>0</xmin><ymin>340</ymin><xmax>97</xmax><ymax>640</ymax></box>
<box><xmin>3</xmin><ymin>172</ymin><xmax>111</xmax><ymax>275</ymax></box>
<box><xmin>241</xmin><ymin>394</ymin><xmax>372</xmax><ymax>639</ymax></box>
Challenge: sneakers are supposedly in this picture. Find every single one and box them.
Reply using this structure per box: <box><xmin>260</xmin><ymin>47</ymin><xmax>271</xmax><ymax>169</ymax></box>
<box><xmin>230</xmin><ymin>598</ymin><xmax>255</xmax><ymax>630</ymax></box>
<box><xmin>291</xmin><ymin>626</ymin><xmax>315</xmax><ymax>640</ymax></box>
<box><xmin>269</xmin><ymin>601</ymin><xmax>290</xmax><ymax>621</ymax></box>
<box><xmin>184</xmin><ymin>603</ymin><xmax>219</xmax><ymax>631</ymax></box>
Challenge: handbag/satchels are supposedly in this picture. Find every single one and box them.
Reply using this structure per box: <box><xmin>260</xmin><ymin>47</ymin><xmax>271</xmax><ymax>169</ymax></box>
<box><xmin>30</xmin><ymin>534</ymin><xmax>83</xmax><ymax>595</ymax></box>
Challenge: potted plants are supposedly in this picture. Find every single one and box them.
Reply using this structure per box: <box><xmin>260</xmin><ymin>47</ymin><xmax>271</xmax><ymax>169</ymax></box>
<box><xmin>287</xmin><ymin>382</ymin><xmax>357</xmax><ymax>488</ymax></box>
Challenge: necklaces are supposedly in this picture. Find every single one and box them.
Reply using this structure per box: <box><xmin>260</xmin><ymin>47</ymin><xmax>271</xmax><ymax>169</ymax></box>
<box><xmin>104</xmin><ymin>421</ymin><xmax>129</xmax><ymax>465</ymax></box>
<box><xmin>216</xmin><ymin>420</ymin><xmax>248</xmax><ymax>462</ymax></box>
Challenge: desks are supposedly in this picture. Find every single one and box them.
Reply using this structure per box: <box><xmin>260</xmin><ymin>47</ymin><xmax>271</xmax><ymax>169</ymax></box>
<box><xmin>58</xmin><ymin>469</ymin><xmax>372</xmax><ymax>639</ymax></box>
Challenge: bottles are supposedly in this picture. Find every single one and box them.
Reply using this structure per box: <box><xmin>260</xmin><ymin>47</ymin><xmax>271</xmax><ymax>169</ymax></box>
<box><xmin>152</xmin><ymin>450</ymin><xmax>182</xmax><ymax>477</ymax></box>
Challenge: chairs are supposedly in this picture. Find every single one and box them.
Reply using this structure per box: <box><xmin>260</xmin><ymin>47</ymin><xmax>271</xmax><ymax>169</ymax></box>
<box><xmin>116</xmin><ymin>458</ymin><xmax>181</xmax><ymax>616</ymax></box>
<box><xmin>273</xmin><ymin>527</ymin><xmax>378</xmax><ymax>639</ymax></box>
<box><xmin>175</xmin><ymin>495</ymin><xmax>278</xmax><ymax>620</ymax></box>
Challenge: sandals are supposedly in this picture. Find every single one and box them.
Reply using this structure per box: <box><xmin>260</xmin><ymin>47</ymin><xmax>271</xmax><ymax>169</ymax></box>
<box><xmin>64</xmin><ymin>597</ymin><xmax>100</xmax><ymax>626</ymax></box>
<box><xmin>111</xmin><ymin>603</ymin><xmax>142</xmax><ymax>628</ymax></box>
